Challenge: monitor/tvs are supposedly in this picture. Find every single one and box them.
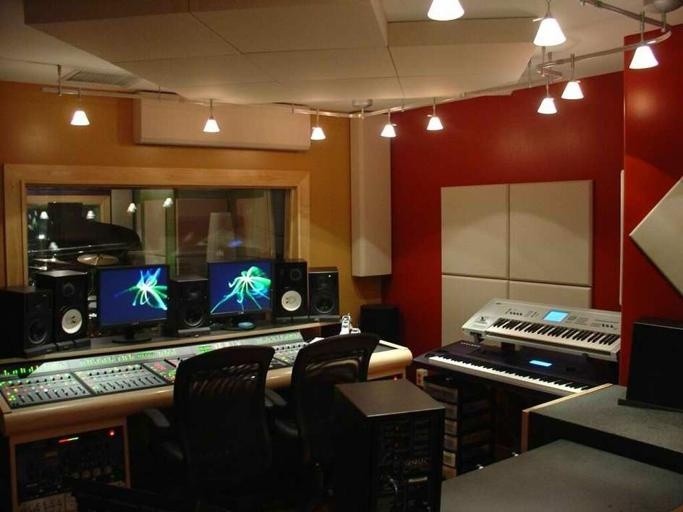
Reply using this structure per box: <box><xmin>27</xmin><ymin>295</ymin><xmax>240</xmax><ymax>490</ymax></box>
<box><xmin>95</xmin><ymin>263</ymin><xmax>171</xmax><ymax>344</ymax></box>
<box><xmin>205</xmin><ymin>260</ymin><xmax>274</xmax><ymax>331</ymax></box>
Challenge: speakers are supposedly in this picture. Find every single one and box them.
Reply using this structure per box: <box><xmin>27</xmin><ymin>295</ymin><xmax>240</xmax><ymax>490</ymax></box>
<box><xmin>1</xmin><ymin>284</ymin><xmax>58</xmax><ymax>358</ymax></box>
<box><xmin>309</xmin><ymin>266</ymin><xmax>339</xmax><ymax>319</ymax></box>
<box><xmin>273</xmin><ymin>258</ymin><xmax>309</xmax><ymax>321</ymax></box>
<box><xmin>171</xmin><ymin>274</ymin><xmax>211</xmax><ymax>338</ymax></box>
<box><xmin>36</xmin><ymin>270</ymin><xmax>92</xmax><ymax>351</ymax></box>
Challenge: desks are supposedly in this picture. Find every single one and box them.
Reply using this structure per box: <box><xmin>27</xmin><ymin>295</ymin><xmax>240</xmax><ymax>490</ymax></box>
<box><xmin>438</xmin><ymin>439</ymin><xmax>683</xmax><ymax>512</ymax></box>
<box><xmin>0</xmin><ymin>322</ymin><xmax>412</xmax><ymax>512</ymax></box>
<box><xmin>522</xmin><ymin>382</ymin><xmax>683</xmax><ymax>474</ymax></box>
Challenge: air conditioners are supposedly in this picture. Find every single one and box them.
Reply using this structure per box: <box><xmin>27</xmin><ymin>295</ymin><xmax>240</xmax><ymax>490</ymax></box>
<box><xmin>133</xmin><ymin>91</ymin><xmax>312</xmax><ymax>152</ymax></box>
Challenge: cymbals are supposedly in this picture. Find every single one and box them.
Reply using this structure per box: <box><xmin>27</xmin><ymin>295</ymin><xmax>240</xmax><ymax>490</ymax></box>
<box><xmin>77</xmin><ymin>253</ymin><xmax>119</xmax><ymax>266</ymax></box>
<box><xmin>34</xmin><ymin>256</ymin><xmax>70</xmax><ymax>265</ymax></box>
<box><xmin>29</xmin><ymin>265</ymin><xmax>49</xmax><ymax>270</ymax></box>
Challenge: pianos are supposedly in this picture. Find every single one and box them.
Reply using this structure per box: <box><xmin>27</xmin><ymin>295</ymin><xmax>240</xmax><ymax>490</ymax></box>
<box><xmin>413</xmin><ymin>338</ymin><xmax>619</xmax><ymax>399</ymax></box>
<box><xmin>461</xmin><ymin>297</ymin><xmax>621</xmax><ymax>362</ymax></box>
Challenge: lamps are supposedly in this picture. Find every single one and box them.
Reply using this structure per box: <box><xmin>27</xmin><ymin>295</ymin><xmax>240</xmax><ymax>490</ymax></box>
<box><xmin>629</xmin><ymin>6</ymin><xmax>659</xmax><ymax>70</ymax></box>
<box><xmin>311</xmin><ymin>104</ymin><xmax>327</xmax><ymax>141</ymax></box>
<box><xmin>561</xmin><ymin>53</ymin><xmax>584</xmax><ymax>104</ymax></box>
<box><xmin>537</xmin><ymin>79</ymin><xmax>558</xmax><ymax>114</ymax></box>
<box><xmin>426</xmin><ymin>98</ymin><xmax>444</xmax><ymax>132</ymax></box>
<box><xmin>203</xmin><ymin>99</ymin><xmax>222</xmax><ymax>134</ymax></box>
<box><xmin>70</xmin><ymin>88</ymin><xmax>92</xmax><ymax>126</ymax></box>
<box><xmin>532</xmin><ymin>0</ymin><xmax>567</xmax><ymax>48</ymax></box>
<box><xmin>381</xmin><ymin>105</ymin><xmax>397</xmax><ymax>138</ymax></box>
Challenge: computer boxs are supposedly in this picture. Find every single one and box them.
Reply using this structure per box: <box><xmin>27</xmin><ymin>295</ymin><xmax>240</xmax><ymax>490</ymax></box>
<box><xmin>334</xmin><ymin>378</ymin><xmax>446</xmax><ymax>511</ymax></box>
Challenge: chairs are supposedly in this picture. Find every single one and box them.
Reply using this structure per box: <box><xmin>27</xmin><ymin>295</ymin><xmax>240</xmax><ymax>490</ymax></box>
<box><xmin>70</xmin><ymin>345</ymin><xmax>276</xmax><ymax>512</ymax></box>
<box><xmin>271</xmin><ymin>334</ymin><xmax>381</xmax><ymax>511</ymax></box>
